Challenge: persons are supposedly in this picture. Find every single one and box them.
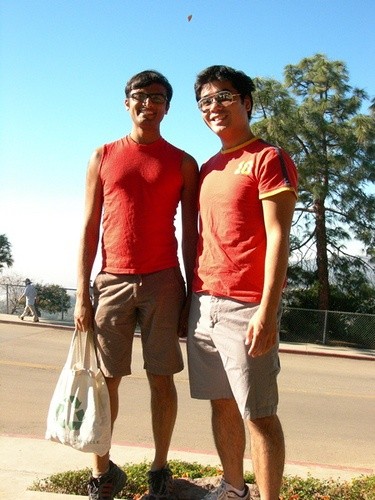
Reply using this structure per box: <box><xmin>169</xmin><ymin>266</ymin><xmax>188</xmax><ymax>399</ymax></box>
<box><xmin>188</xmin><ymin>65</ymin><xmax>298</xmax><ymax>500</ymax></box>
<box><xmin>18</xmin><ymin>278</ymin><xmax>39</xmax><ymax>322</ymax></box>
<box><xmin>74</xmin><ymin>71</ymin><xmax>201</xmax><ymax>500</ymax></box>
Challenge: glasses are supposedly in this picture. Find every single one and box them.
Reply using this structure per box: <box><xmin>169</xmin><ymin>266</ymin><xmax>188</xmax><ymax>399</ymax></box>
<box><xmin>127</xmin><ymin>92</ymin><xmax>169</xmax><ymax>106</ymax></box>
<box><xmin>197</xmin><ymin>91</ymin><xmax>244</xmax><ymax>114</ymax></box>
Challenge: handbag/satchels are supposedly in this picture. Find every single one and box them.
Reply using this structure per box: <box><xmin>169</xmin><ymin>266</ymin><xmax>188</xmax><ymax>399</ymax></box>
<box><xmin>44</xmin><ymin>326</ymin><xmax>113</xmax><ymax>459</ymax></box>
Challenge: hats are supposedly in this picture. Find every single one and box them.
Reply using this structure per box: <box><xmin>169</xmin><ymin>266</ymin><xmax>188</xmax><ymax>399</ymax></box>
<box><xmin>23</xmin><ymin>278</ymin><xmax>32</xmax><ymax>284</ymax></box>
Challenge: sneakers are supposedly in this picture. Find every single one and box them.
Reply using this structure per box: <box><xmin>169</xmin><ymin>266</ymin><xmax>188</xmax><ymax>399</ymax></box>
<box><xmin>147</xmin><ymin>464</ymin><xmax>177</xmax><ymax>500</ymax></box>
<box><xmin>87</xmin><ymin>460</ymin><xmax>127</xmax><ymax>500</ymax></box>
<box><xmin>200</xmin><ymin>480</ymin><xmax>251</xmax><ymax>500</ymax></box>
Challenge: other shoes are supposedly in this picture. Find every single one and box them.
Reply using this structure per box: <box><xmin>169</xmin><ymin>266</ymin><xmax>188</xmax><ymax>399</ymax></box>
<box><xmin>32</xmin><ymin>320</ymin><xmax>39</xmax><ymax>322</ymax></box>
<box><xmin>18</xmin><ymin>315</ymin><xmax>24</xmax><ymax>320</ymax></box>
